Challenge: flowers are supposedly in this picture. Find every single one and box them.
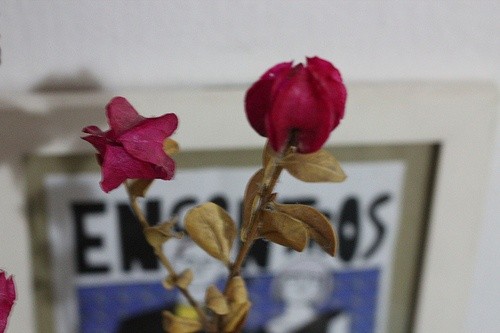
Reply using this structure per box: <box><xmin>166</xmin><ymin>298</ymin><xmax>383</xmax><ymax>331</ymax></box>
<box><xmin>0</xmin><ymin>52</ymin><xmax>353</xmax><ymax>333</ymax></box>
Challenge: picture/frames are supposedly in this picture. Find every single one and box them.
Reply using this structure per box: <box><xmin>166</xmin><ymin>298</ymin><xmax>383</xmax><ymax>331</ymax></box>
<box><xmin>21</xmin><ymin>136</ymin><xmax>445</xmax><ymax>333</ymax></box>
